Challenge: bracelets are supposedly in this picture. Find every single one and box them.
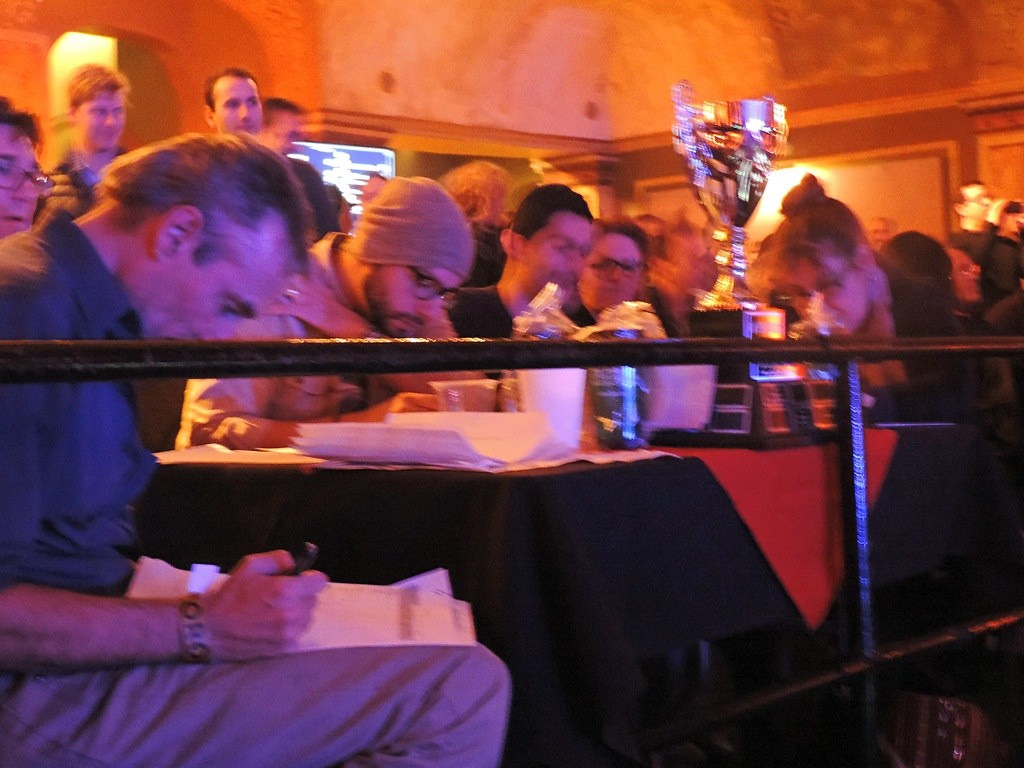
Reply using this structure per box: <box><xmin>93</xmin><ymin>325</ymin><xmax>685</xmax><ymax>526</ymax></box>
<box><xmin>182</xmin><ymin>595</ymin><xmax>210</xmax><ymax>665</ymax></box>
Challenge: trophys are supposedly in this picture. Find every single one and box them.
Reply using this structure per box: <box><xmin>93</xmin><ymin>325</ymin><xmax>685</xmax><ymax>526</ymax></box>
<box><xmin>671</xmin><ymin>78</ymin><xmax>789</xmax><ymax>337</ymax></box>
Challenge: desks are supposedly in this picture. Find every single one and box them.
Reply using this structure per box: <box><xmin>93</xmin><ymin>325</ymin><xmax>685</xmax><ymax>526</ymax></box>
<box><xmin>137</xmin><ymin>421</ymin><xmax>993</xmax><ymax>768</ymax></box>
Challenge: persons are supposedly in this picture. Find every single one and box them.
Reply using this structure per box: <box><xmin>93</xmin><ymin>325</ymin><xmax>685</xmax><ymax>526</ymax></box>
<box><xmin>361</xmin><ymin>173</ymin><xmax>388</xmax><ymax>203</ymax></box>
<box><xmin>175</xmin><ymin>178</ymin><xmax>476</xmax><ymax>448</ymax></box>
<box><xmin>52</xmin><ymin>62</ymin><xmax>130</xmax><ymax>190</ymax></box>
<box><xmin>255</xmin><ymin>96</ymin><xmax>340</xmax><ymax>242</ymax></box>
<box><xmin>324</xmin><ymin>183</ymin><xmax>354</xmax><ymax>233</ymax></box>
<box><xmin>0</xmin><ymin>130</ymin><xmax>511</xmax><ymax>768</ymax></box>
<box><xmin>203</xmin><ymin>68</ymin><xmax>262</xmax><ymax>137</ymax></box>
<box><xmin>0</xmin><ymin>95</ymin><xmax>74</xmax><ymax>239</ymax></box>
<box><xmin>436</xmin><ymin>158</ymin><xmax>1024</xmax><ymax>768</ymax></box>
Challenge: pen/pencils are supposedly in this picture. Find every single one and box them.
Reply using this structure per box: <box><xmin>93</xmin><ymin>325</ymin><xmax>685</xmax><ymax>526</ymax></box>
<box><xmin>290</xmin><ymin>542</ymin><xmax>318</xmax><ymax>575</ymax></box>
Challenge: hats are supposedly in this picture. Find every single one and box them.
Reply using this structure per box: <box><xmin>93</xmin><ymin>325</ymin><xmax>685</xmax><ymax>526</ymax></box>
<box><xmin>351</xmin><ymin>177</ymin><xmax>478</xmax><ymax>278</ymax></box>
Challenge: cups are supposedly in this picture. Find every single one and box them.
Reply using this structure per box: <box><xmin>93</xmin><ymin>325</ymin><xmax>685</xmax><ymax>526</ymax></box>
<box><xmin>429</xmin><ymin>326</ymin><xmax>637</xmax><ymax>450</ymax></box>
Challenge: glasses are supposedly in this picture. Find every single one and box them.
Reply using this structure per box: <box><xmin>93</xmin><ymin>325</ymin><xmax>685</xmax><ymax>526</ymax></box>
<box><xmin>585</xmin><ymin>256</ymin><xmax>645</xmax><ymax>272</ymax></box>
<box><xmin>0</xmin><ymin>160</ymin><xmax>56</xmax><ymax>199</ymax></box>
<box><xmin>405</xmin><ymin>267</ymin><xmax>470</xmax><ymax>311</ymax></box>
<box><xmin>779</xmin><ymin>266</ymin><xmax>851</xmax><ymax>308</ymax></box>
